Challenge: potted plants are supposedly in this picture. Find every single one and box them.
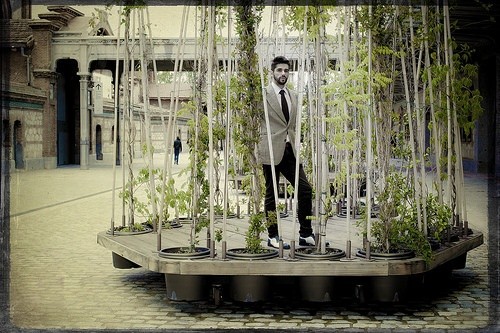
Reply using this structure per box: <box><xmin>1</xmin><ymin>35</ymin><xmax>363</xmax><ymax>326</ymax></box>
<box><xmin>89</xmin><ymin>5</ymin><xmax>469</xmax><ymax>303</ymax></box>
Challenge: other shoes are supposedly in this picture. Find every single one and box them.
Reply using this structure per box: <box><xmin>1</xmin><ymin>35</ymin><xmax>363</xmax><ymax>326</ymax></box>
<box><xmin>299</xmin><ymin>233</ymin><xmax>329</xmax><ymax>246</ymax></box>
<box><xmin>267</xmin><ymin>236</ymin><xmax>291</xmax><ymax>249</ymax></box>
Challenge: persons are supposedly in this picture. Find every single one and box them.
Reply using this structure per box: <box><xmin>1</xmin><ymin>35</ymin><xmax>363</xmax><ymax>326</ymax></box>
<box><xmin>174</xmin><ymin>136</ymin><xmax>182</xmax><ymax>165</ymax></box>
<box><xmin>256</xmin><ymin>56</ymin><xmax>318</xmax><ymax>249</ymax></box>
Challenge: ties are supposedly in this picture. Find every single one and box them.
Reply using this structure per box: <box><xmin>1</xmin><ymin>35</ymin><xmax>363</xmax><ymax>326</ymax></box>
<box><xmin>278</xmin><ymin>90</ymin><xmax>290</xmax><ymax>124</ymax></box>
